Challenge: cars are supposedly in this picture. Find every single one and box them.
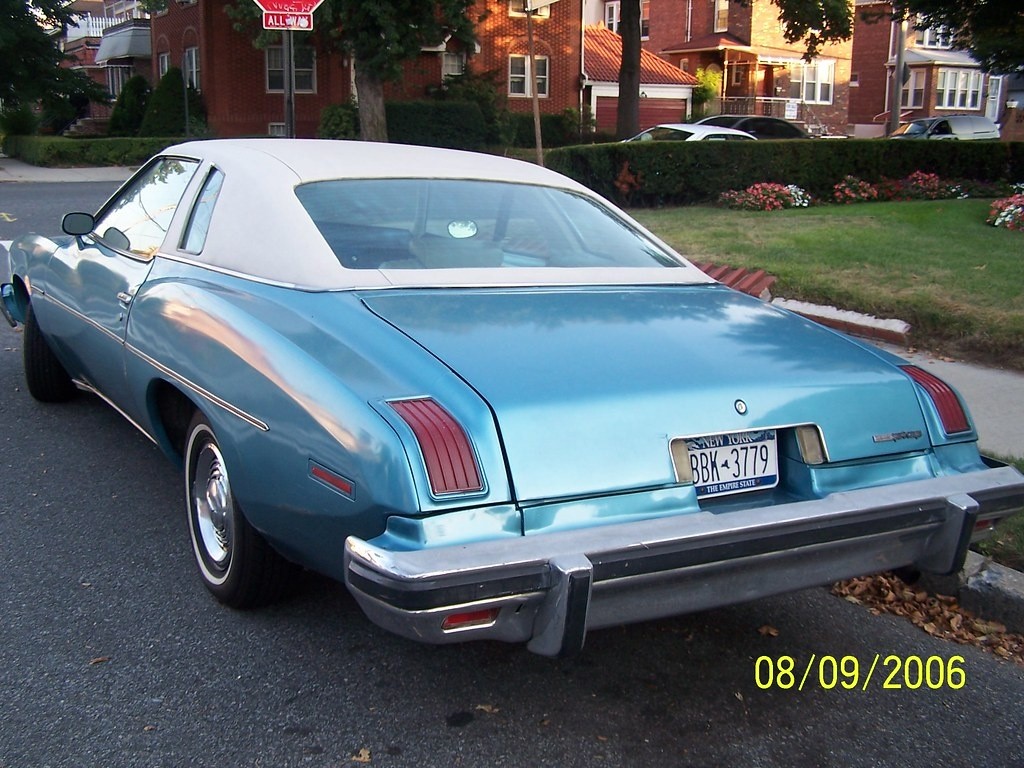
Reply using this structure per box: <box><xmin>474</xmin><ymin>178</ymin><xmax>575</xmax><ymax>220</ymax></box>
<box><xmin>617</xmin><ymin>125</ymin><xmax>758</xmax><ymax>144</ymax></box>
<box><xmin>694</xmin><ymin>114</ymin><xmax>814</xmax><ymax>139</ymax></box>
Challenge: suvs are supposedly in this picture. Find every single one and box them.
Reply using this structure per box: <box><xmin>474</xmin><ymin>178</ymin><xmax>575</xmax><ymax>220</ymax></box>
<box><xmin>887</xmin><ymin>115</ymin><xmax>1002</xmax><ymax>137</ymax></box>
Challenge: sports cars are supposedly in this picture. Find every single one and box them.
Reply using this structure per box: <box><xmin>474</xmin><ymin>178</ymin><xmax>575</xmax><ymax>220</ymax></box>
<box><xmin>0</xmin><ymin>138</ymin><xmax>1024</xmax><ymax>664</ymax></box>
